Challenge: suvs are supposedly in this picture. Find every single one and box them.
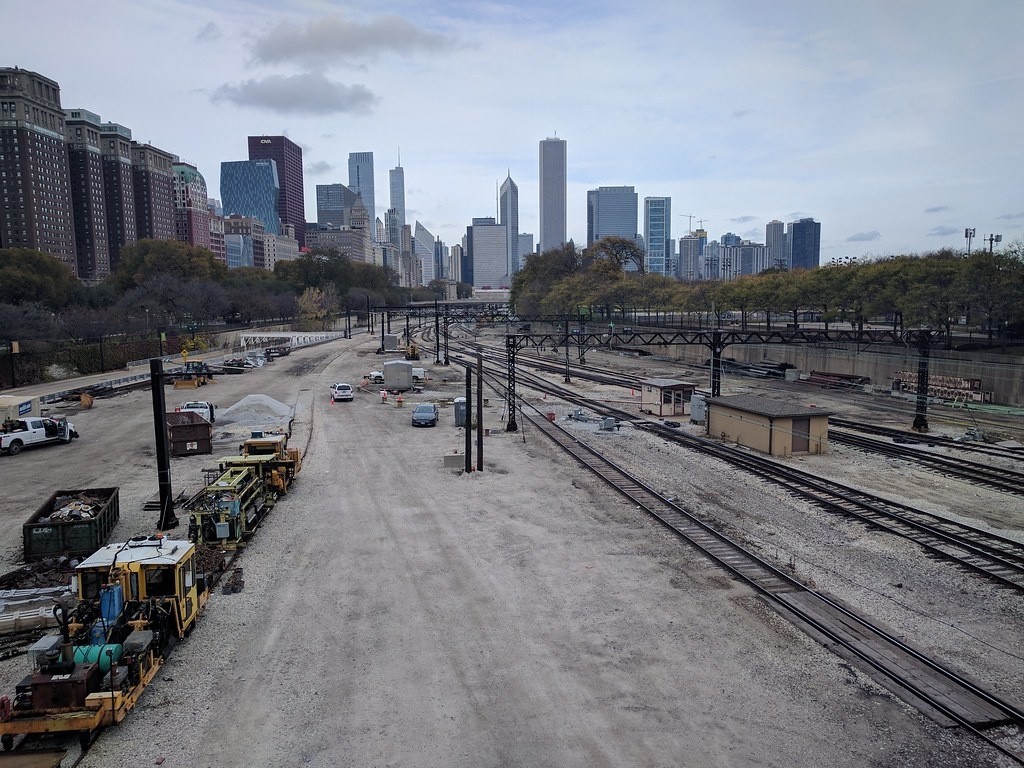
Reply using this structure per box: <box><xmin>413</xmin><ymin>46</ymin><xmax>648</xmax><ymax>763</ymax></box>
<box><xmin>329</xmin><ymin>382</ymin><xmax>354</xmax><ymax>402</ymax></box>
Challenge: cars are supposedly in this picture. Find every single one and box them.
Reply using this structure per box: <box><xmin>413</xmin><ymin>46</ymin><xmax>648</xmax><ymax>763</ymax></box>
<box><xmin>411</xmin><ymin>404</ymin><xmax>439</xmax><ymax>427</ymax></box>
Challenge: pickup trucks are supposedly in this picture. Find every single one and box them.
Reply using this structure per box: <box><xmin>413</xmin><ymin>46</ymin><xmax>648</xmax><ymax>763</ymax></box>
<box><xmin>0</xmin><ymin>416</ymin><xmax>75</xmax><ymax>455</ymax></box>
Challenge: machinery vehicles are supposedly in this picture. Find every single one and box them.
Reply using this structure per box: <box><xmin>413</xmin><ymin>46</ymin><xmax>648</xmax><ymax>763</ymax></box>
<box><xmin>173</xmin><ymin>359</ymin><xmax>213</xmax><ymax>390</ymax></box>
<box><xmin>405</xmin><ymin>344</ymin><xmax>419</xmax><ymax>360</ymax></box>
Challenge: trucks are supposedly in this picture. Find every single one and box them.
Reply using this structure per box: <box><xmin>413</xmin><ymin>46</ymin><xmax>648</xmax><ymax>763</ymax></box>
<box><xmin>173</xmin><ymin>398</ymin><xmax>217</xmax><ymax>426</ymax></box>
<box><xmin>368</xmin><ymin>367</ymin><xmax>426</xmax><ymax>384</ymax></box>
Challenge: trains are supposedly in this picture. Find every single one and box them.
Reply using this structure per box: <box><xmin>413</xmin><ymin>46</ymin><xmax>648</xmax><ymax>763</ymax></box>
<box><xmin>0</xmin><ymin>414</ymin><xmax>302</xmax><ymax>767</ymax></box>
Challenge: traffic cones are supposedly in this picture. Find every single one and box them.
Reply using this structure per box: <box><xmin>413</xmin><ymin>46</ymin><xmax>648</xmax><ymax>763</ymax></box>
<box><xmin>330</xmin><ymin>397</ymin><xmax>334</xmax><ymax>405</ymax></box>
